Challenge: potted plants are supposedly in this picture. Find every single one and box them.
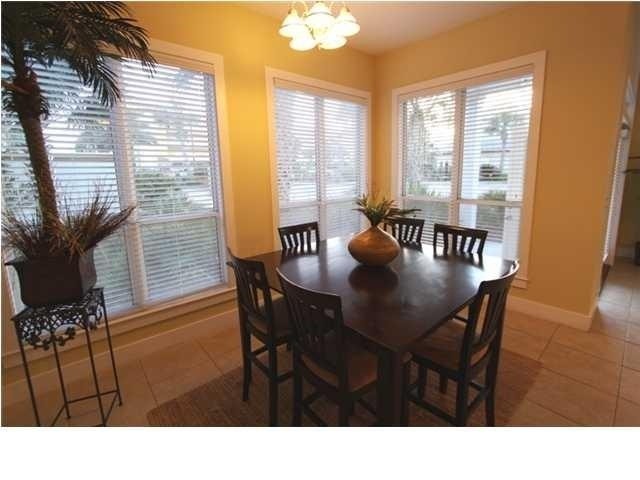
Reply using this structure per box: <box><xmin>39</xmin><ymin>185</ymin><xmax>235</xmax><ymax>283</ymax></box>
<box><xmin>347</xmin><ymin>187</ymin><xmax>423</xmax><ymax>265</ymax></box>
<box><xmin>1</xmin><ymin>1</ymin><xmax>157</xmax><ymax>307</ymax></box>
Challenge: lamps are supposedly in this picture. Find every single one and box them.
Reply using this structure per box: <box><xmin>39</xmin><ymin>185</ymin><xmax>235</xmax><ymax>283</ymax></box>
<box><xmin>276</xmin><ymin>1</ymin><xmax>360</xmax><ymax>53</ymax></box>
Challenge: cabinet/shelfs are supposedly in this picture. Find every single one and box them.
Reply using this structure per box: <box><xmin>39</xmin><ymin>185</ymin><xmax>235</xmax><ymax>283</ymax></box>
<box><xmin>10</xmin><ymin>287</ymin><xmax>123</xmax><ymax>427</ymax></box>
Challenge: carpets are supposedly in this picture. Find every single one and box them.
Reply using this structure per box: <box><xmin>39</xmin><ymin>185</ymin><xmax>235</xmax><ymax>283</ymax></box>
<box><xmin>146</xmin><ymin>320</ymin><xmax>544</xmax><ymax>427</ymax></box>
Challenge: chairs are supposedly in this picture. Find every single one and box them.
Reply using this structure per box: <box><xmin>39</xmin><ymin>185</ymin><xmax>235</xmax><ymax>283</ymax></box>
<box><xmin>225</xmin><ymin>216</ymin><xmax>521</xmax><ymax>427</ymax></box>
